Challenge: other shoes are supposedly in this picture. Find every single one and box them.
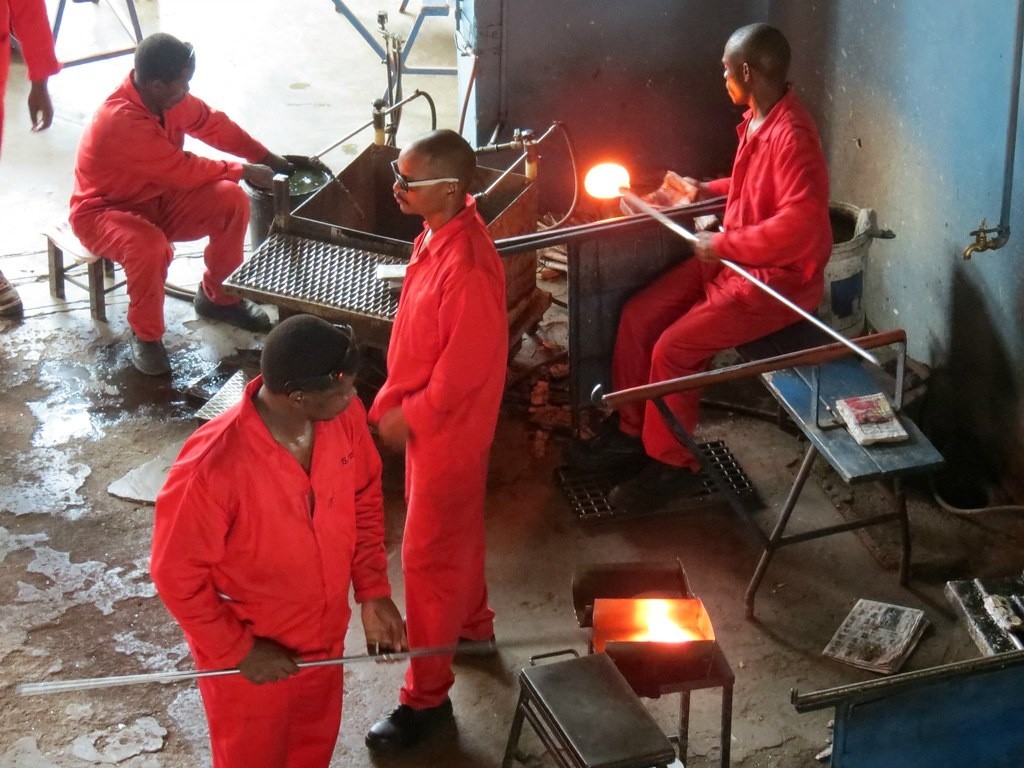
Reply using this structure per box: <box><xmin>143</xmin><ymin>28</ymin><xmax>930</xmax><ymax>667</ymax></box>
<box><xmin>1</xmin><ymin>285</ymin><xmax>24</xmax><ymax>317</ymax></box>
<box><xmin>365</xmin><ymin>696</ymin><xmax>453</xmax><ymax>749</ymax></box>
<box><xmin>455</xmin><ymin>636</ymin><xmax>498</xmax><ymax>662</ymax></box>
<box><xmin>128</xmin><ymin>329</ymin><xmax>171</xmax><ymax>375</ymax></box>
<box><xmin>194</xmin><ymin>282</ymin><xmax>269</xmax><ymax>329</ymax></box>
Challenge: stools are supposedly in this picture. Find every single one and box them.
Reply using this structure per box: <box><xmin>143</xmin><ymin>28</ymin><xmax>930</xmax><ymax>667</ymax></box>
<box><xmin>39</xmin><ymin>219</ymin><xmax>127</xmax><ymax>321</ymax></box>
<box><xmin>502</xmin><ymin>647</ymin><xmax>678</xmax><ymax>768</ymax></box>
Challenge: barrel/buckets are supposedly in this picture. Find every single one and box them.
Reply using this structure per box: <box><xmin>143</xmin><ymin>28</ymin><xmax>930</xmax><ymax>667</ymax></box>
<box><xmin>241</xmin><ymin>156</ymin><xmax>331</xmax><ymax>252</ymax></box>
<box><xmin>817</xmin><ymin>200</ymin><xmax>873</xmax><ymax>341</ymax></box>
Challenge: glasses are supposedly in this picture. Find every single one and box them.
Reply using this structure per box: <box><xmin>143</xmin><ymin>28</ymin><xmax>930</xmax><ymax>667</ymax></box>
<box><xmin>391</xmin><ymin>160</ymin><xmax>461</xmax><ymax>192</ymax></box>
<box><xmin>284</xmin><ymin>324</ymin><xmax>359</xmax><ymax>390</ymax></box>
<box><xmin>174</xmin><ymin>41</ymin><xmax>195</xmax><ymax>69</ymax></box>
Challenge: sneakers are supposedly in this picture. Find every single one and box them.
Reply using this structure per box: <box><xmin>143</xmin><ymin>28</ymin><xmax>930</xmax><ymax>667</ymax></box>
<box><xmin>567</xmin><ymin>425</ymin><xmax>645</xmax><ymax>470</ymax></box>
<box><xmin>610</xmin><ymin>460</ymin><xmax>701</xmax><ymax>510</ymax></box>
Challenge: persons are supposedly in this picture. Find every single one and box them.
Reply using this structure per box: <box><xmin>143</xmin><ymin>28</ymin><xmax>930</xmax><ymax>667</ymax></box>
<box><xmin>364</xmin><ymin>128</ymin><xmax>508</xmax><ymax>751</ymax></box>
<box><xmin>0</xmin><ymin>0</ymin><xmax>62</xmax><ymax>315</ymax></box>
<box><xmin>150</xmin><ymin>315</ymin><xmax>409</xmax><ymax>768</ymax></box>
<box><xmin>567</xmin><ymin>22</ymin><xmax>833</xmax><ymax>511</ymax></box>
<box><xmin>70</xmin><ymin>33</ymin><xmax>289</xmax><ymax>375</ymax></box>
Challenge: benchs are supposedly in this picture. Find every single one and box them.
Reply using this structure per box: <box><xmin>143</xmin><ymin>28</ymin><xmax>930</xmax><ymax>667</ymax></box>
<box><xmin>732</xmin><ymin>316</ymin><xmax>945</xmax><ymax>623</ymax></box>
